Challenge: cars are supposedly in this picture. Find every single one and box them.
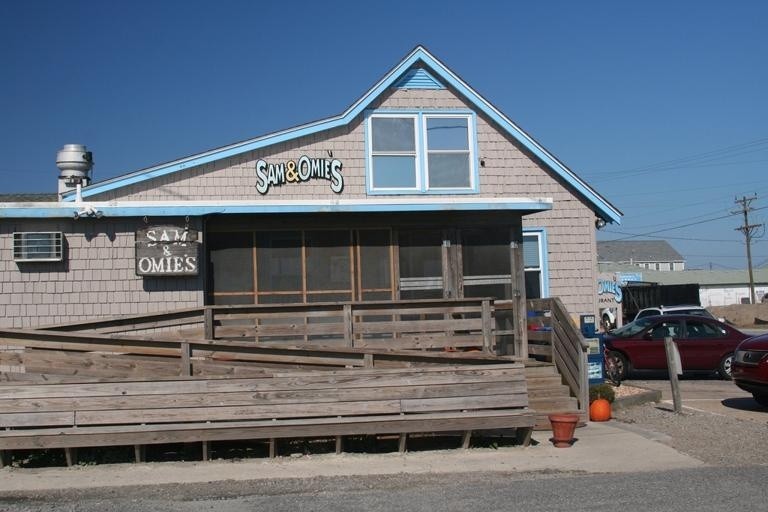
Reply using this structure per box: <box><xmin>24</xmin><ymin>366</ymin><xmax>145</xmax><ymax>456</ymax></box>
<box><xmin>732</xmin><ymin>333</ymin><xmax>767</xmax><ymax>409</ymax></box>
<box><xmin>603</xmin><ymin>313</ymin><xmax>759</xmax><ymax>380</ymax></box>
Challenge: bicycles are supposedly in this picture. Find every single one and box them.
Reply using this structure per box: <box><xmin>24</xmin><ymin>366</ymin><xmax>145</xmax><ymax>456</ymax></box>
<box><xmin>603</xmin><ymin>344</ymin><xmax>620</xmax><ymax>385</ymax></box>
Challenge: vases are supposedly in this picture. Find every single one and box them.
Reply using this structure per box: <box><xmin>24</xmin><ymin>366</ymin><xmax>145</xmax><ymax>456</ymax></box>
<box><xmin>548</xmin><ymin>414</ymin><xmax>578</xmax><ymax>448</ymax></box>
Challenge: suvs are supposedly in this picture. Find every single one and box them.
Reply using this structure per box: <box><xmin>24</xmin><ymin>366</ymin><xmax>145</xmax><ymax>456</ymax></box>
<box><xmin>631</xmin><ymin>303</ymin><xmax>722</xmax><ymax>322</ymax></box>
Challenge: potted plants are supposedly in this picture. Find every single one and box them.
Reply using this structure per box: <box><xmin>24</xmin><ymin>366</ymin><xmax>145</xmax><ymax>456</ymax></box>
<box><xmin>589</xmin><ymin>385</ymin><xmax>615</xmax><ymax>421</ymax></box>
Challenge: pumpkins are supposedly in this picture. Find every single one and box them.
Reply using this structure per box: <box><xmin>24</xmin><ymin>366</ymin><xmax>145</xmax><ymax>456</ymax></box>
<box><xmin>590</xmin><ymin>391</ymin><xmax>612</xmax><ymax>421</ymax></box>
